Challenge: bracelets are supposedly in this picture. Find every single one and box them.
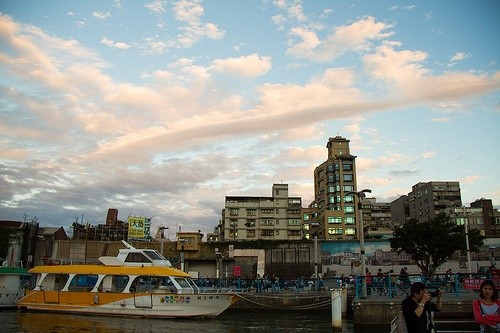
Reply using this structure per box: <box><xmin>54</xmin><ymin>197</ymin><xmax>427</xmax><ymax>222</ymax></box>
<box><xmin>419</xmin><ymin>303</ymin><xmax>425</xmax><ymax>306</ymax></box>
<box><xmin>437</xmin><ymin>301</ymin><xmax>443</xmax><ymax>303</ymax></box>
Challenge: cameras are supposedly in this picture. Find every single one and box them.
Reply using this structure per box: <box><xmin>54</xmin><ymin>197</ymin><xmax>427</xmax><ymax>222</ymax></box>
<box><xmin>430</xmin><ymin>292</ymin><xmax>438</xmax><ymax>297</ymax></box>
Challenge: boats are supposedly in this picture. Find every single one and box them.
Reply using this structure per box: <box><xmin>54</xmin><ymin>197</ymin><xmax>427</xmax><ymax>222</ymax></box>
<box><xmin>16</xmin><ymin>239</ymin><xmax>250</xmax><ymax>320</ymax></box>
<box><xmin>0</xmin><ymin>265</ymin><xmax>32</xmax><ymax>309</ymax></box>
<box><xmin>233</xmin><ymin>294</ymin><xmax>356</xmax><ymax>318</ymax></box>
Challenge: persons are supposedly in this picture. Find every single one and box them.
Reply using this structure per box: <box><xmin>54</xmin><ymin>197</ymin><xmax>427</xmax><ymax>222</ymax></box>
<box><xmin>444</xmin><ymin>269</ymin><xmax>457</xmax><ymax>293</ymax></box>
<box><xmin>364</xmin><ymin>267</ymin><xmax>411</xmax><ymax>298</ymax></box>
<box><xmin>401</xmin><ymin>282</ymin><xmax>443</xmax><ymax>333</ymax></box>
<box><xmin>297</xmin><ymin>269</ymin><xmax>337</xmax><ymax>288</ymax></box>
<box><xmin>472</xmin><ymin>280</ymin><xmax>500</xmax><ymax>333</ymax></box>
<box><xmin>196</xmin><ymin>273</ymin><xmax>278</xmax><ymax>293</ymax></box>
<box><xmin>486</xmin><ymin>266</ymin><xmax>496</xmax><ymax>281</ymax></box>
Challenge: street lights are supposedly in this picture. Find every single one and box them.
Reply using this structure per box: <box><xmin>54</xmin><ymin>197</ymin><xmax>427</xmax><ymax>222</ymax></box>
<box><xmin>345</xmin><ymin>188</ymin><xmax>372</xmax><ymax>300</ymax></box>
